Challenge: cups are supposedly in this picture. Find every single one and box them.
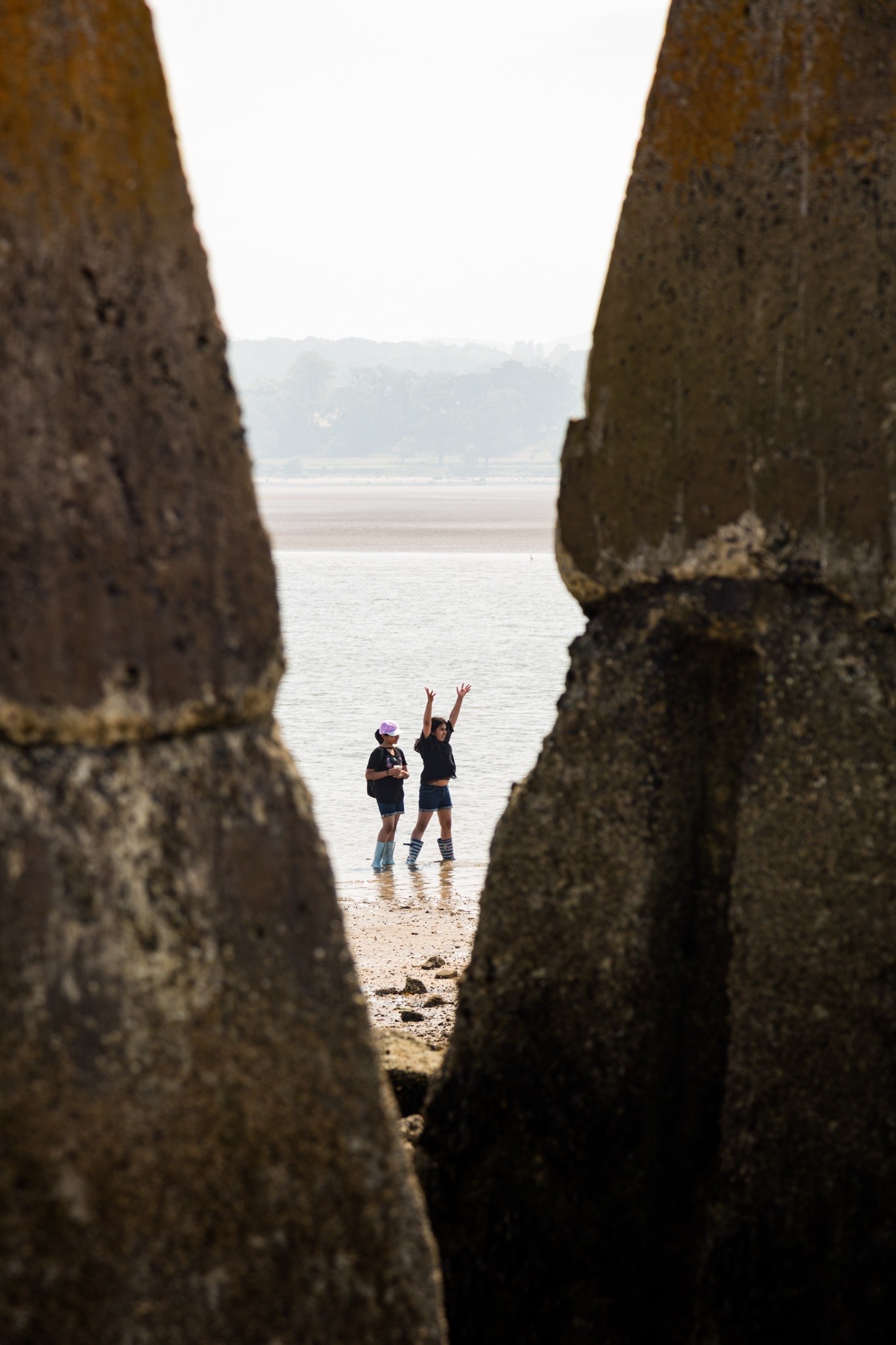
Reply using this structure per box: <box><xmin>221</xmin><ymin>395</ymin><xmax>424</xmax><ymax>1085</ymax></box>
<box><xmin>393</xmin><ymin>765</ymin><xmax>403</xmax><ymax>778</ymax></box>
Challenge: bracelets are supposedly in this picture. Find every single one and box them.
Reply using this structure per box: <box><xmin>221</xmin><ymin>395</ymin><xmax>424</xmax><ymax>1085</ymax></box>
<box><xmin>386</xmin><ymin>770</ymin><xmax>389</xmax><ymax>777</ymax></box>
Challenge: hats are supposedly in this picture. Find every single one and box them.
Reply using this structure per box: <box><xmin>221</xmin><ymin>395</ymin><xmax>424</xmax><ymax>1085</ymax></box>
<box><xmin>379</xmin><ymin>720</ymin><xmax>404</xmax><ymax>736</ymax></box>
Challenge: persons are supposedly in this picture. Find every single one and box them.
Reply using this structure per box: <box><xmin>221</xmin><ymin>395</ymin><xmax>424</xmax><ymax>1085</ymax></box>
<box><xmin>365</xmin><ymin>720</ymin><xmax>409</xmax><ymax>868</ymax></box>
<box><xmin>406</xmin><ymin>682</ymin><xmax>472</xmax><ymax>864</ymax></box>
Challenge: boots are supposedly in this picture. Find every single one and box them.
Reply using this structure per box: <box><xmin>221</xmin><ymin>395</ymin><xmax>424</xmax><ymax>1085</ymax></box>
<box><xmin>437</xmin><ymin>838</ymin><xmax>456</xmax><ymax>860</ymax></box>
<box><xmin>403</xmin><ymin>838</ymin><xmax>424</xmax><ymax>865</ymax></box>
<box><xmin>371</xmin><ymin>841</ymin><xmax>387</xmax><ymax>868</ymax></box>
<box><xmin>382</xmin><ymin>841</ymin><xmax>396</xmax><ymax>864</ymax></box>
